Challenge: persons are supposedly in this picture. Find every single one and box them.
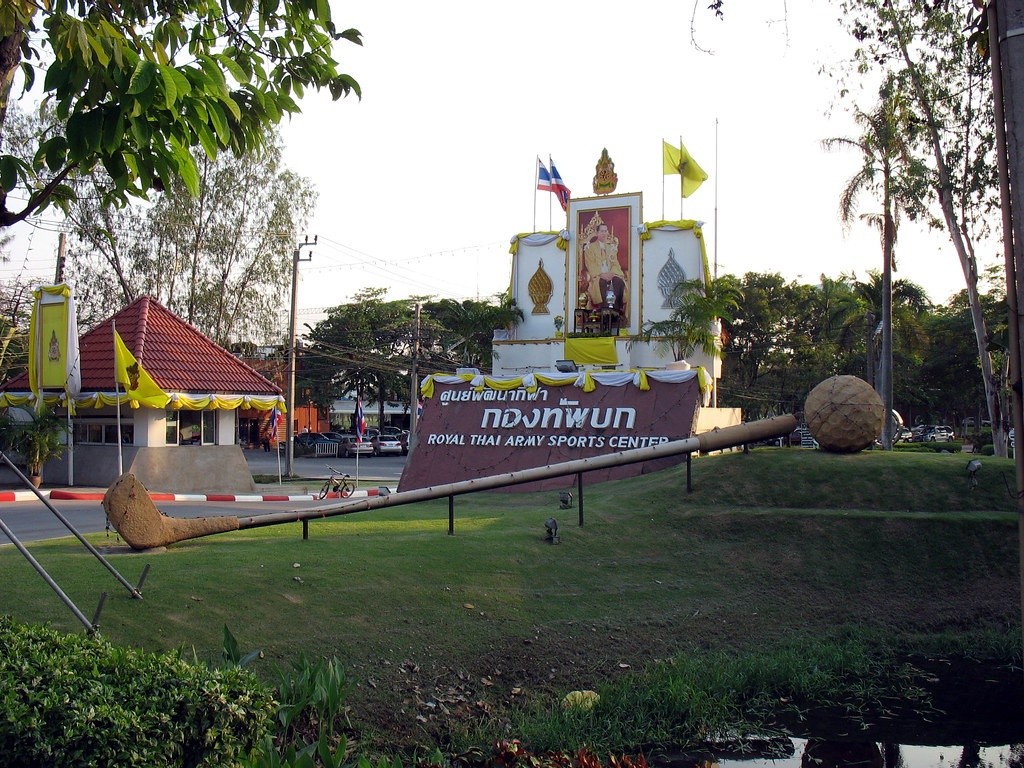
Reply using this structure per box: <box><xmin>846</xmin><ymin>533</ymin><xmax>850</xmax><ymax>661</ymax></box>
<box><xmin>301</xmin><ymin>426</ymin><xmax>308</xmax><ymax>433</ymax></box>
<box><xmin>583</xmin><ymin>224</ymin><xmax>625</xmax><ymax>311</ymax></box>
<box><xmin>262</xmin><ymin>428</ymin><xmax>270</xmax><ymax>452</ymax></box>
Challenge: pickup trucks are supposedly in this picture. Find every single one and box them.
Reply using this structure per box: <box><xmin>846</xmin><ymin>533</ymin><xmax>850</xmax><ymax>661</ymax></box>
<box><xmin>962</xmin><ymin>417</ymin><xmax>992</xmax><ymax>428</ymax></box>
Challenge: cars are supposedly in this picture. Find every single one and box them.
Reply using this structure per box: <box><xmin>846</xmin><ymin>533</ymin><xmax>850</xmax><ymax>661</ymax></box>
<box><xmin>339</xmin><ymin>436</ymin><xmax>374</xmax><ymax>458</ymax></box>
<box><xmin>363</xmin><ymin>425</ymin><xmax>410</xmax><ymax>451</ymax></box>
<box><xmin>370</xmin><ymin>435</ymin><xmax>403</xmax><ymax>456</ymax></box>
<box><xmin>295</xmin><ymin>432</ymin><xmax>343</xmax><ymax>451</ymax></box>
<box><xmin>766</xmin><ymin>427</ymin><xmax>811</xmax><ymax>446</ymax></box>
<box><xmin>892</xmin><ymin>424</ymin><xmax>955</xmax><ymax>443</ymax></box>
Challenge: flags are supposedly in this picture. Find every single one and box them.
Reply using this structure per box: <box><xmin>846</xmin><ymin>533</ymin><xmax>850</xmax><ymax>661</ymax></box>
<box><xmin>115</xmin><ymin>329</ymin><xmax>171</xmax><ymax>409</ymax></box>
<box><xmin>550</xmin><ymin>159</ymin><xmax>571</xmax><ymax>212</ymax></box>
<box><xmin>271</xmin><ymin>405</ymin><xmax>282</xmax><ymax>442</ymax></box>
<box><xmin>675</xmin><ymin>143</ymin><xmax>708</xmax><ymax>198</ymax></box>
<box><xmin>355</xmin><ymin>395</ymin><xmax>367</xmax><ymax>443</ymax></box>
<box><xmin>537</xmin><ymin>158</ymin><xmax>550</xmax><ymax>191</ymax></box>
<box><xmin>663</xmin><ymin>142</ymin><xmax>681</xmax><ymax>175</ymax></box>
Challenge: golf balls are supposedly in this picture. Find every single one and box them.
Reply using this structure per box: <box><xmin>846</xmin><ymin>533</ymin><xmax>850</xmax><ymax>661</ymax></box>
<box><xmin>804</xmin><ymin>375</ymin><xmax>885</xmax><ymax>452</ymax></box>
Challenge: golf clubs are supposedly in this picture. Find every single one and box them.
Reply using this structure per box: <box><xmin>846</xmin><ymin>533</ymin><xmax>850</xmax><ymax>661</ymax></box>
<box><xmin>102</xmin><ymin>413</ymin><xmax>798</xmax><ymax>551</ymax></box>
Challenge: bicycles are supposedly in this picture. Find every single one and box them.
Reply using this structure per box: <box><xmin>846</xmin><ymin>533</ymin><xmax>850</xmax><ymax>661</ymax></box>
<box><xmin>319</xmin><ymin>464</ymin><xmax>357</xmax><ymax>500</ymax></box>
